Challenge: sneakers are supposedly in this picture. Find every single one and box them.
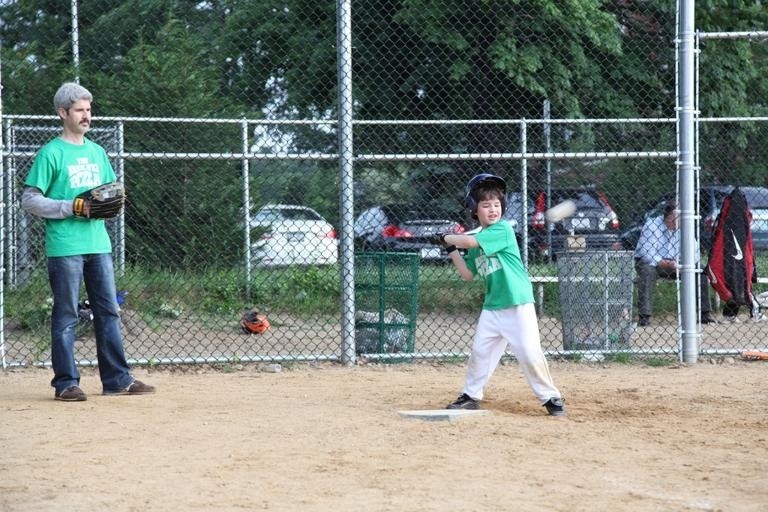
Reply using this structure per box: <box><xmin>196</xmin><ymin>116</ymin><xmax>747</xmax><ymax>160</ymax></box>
<box><xmin>54</xmin><ymin>384</ymin><xmax>87</xmax><ymax>401</ymax></box>
<box><xmin>701</xmin><ymin>314</ymin><xmax>721</xmax><ymax>324</ymax></box>
<box><xmin>545</xmin><ymin>397</ymin><xmax>565</xmax><ymax>416</ymax></box>
<box><xmin>638</xmin><ymin>315</ymin><xmax>649</xmax><ymax>325</ymax></box>
<box><xmin>103</xmin><ymin>379</ymin><xmax>155</xmax><ymax>395</ymax></box>
<box><xmin>445</xmin><ymin>393</ymin><xmax>480</xmax><ymax>409</ymax></box>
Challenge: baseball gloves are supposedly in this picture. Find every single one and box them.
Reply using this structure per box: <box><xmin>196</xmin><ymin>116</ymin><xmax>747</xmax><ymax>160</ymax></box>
<box><xmin>73</xmin><ymin>182</ymin><xmax>123</xmax><ymax>220</ymax></box>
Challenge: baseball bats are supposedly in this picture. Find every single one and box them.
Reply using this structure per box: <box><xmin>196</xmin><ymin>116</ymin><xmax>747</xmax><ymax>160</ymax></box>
<box><xmin>442</xmin><ymin>219</ymin><xmax>518</xmax><ymax>256</ymax></box>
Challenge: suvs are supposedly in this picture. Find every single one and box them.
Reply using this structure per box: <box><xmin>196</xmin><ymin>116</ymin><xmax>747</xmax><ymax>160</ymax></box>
<box><xmin>500</xmin><ymin>182</ymin><xmax>623</xmax><ymax>264</ymax></box>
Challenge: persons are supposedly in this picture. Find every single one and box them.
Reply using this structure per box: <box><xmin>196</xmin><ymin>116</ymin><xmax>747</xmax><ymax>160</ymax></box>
<box><xmin>632</xmin><ymin>201</ymin><xmax>716</xmax><ymax>326</ymax></box>
<box><xmin>18</xmin><ymin>80</ymin><xmax>155</xmax><ymax>402</ymax></box>
<box><xmin>432</xmin><ymin>172</ymin><xmax>566</xmax><ymax>416</ymax></box>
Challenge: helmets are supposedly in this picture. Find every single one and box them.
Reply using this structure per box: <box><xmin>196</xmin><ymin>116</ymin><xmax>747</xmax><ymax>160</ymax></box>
<box><xmin>465</xmin><ymin>173</ymin><xmax>507</xmax><ymax>220</ymax></box>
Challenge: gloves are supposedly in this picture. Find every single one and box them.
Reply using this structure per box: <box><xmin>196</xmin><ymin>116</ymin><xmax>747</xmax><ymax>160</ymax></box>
<box><xmin>429</xmin><ymin>232</ymin><xmax>448</xmax><ymax>245</ymax></box>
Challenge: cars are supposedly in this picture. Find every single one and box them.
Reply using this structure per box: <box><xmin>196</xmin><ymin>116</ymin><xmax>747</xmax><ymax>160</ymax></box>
<box><xmin>352</xmin><ymin>201</ymin><xmax>466</xmax><ymax>264</ymax></box>
<box><xmin>618</xmin><ymin>181</ymin><xmax>767</xmax><ymax>257</ymax></box>
<box><xmin>233</xmin><ymin>204</ymin><xmax>338</xmax><ymax>272</ymax></box>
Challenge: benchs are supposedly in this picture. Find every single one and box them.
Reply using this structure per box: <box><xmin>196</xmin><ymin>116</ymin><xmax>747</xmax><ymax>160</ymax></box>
<box><xmin>527</xmin><ymin>274</ymin><xmax>768</xmax><ymax>321</ymax></box>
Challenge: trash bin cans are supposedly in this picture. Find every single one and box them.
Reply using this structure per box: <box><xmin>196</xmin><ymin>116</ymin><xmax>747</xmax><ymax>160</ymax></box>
<box><xmin>554</xmin><ymin>250</ymin><xmax>635</xmax><ymax>357</ymax></box>
<box><xmin>354</xmin><ymin>252</ymin><xmax>421</xmax><ymax>365</ymax></box>
